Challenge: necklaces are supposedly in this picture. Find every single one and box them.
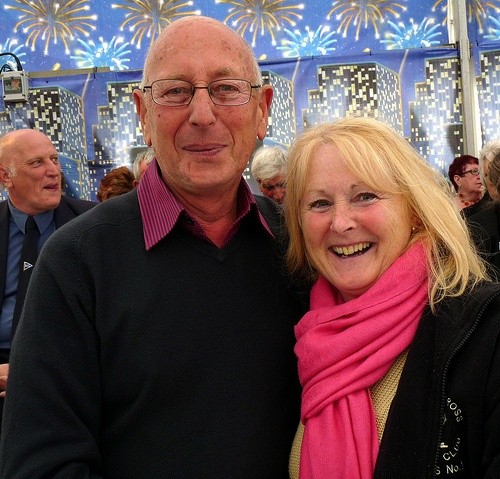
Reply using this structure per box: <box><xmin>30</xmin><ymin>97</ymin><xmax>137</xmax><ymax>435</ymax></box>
<box><xmin>459</xmin><ymin>193</ymin><xmax>480</xmax><ymax>206</ymax></box>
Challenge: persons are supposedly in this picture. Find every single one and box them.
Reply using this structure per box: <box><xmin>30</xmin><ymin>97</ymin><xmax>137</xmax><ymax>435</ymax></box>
<box><xmin>0</xmin><ymin>15</ymin><xmax>311</xmax><ymax>479</ymax></box>
<box><xmin>251</xmin><ymin>146</ymin><xmax>288</xmax><ymax>206</ymax></box>
<box><xmin>449</xmin><ymin>155</ymin><xmax>485</xmax><ymax>212</ymax></box>
<box><xmin>0</xmin><ymin>128</ymin><xmax>100</xmax><ymax>428</ymax></box>
<box><xmin>272</xmin><ymin>115</ymin><xmax>500</xmax><ymax>479</ymax></box>
<box><xmin>459</xmin><ymin>141</ymin><xmax>500</xmax><ymax>285</ymax></box>
<box><xmin>133</xmin><ymin>145</ymin><xmax>155</xmax><ymax>189</ymax></box>
<box><xmin>96</xmin><ymin>167</ymin><xmax>136</xmax><ymax>202</ymax></box>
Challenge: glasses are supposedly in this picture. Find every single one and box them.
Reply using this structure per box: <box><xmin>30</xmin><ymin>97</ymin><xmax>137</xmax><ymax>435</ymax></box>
<box><xmin>462</xmin><ymin>168</ymin><xmax>480</xmax><ymax>175</ymax></box>
<box><xmin>144</xmin><ymin>78</ymin><xmax>264</xmax><ymax>108</ymax></box>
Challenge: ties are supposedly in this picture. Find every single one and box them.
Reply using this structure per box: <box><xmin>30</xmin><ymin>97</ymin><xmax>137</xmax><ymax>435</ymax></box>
<box><xmin>10</xmin><ymin>216</ymin><xmax>40</xmax><ymax>343</ymax></box>
